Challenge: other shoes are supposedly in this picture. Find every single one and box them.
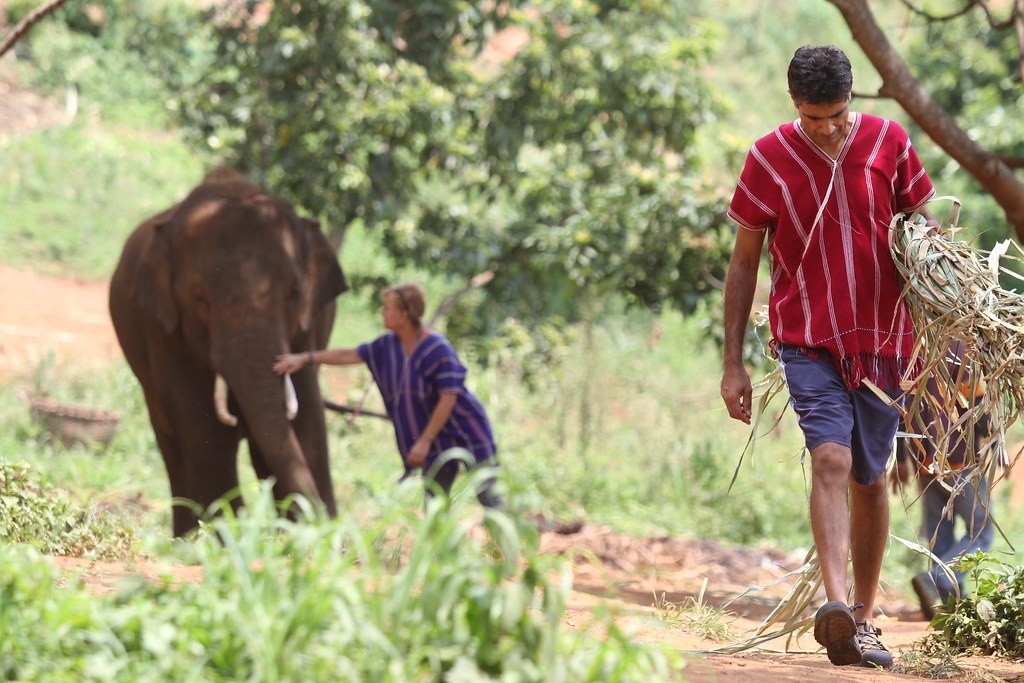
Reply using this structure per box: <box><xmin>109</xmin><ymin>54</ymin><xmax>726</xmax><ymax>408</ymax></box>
<box><xmin>911</xmin><ymin>572</ymin><xmax>949</xmax><ymax>622</ymax></box>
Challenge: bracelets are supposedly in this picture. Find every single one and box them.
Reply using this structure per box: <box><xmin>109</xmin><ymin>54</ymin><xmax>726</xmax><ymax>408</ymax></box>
<box><xmin>306</xmin><ymin>350</ymin><xmax>313</xmax><ymax>366</ymax></box>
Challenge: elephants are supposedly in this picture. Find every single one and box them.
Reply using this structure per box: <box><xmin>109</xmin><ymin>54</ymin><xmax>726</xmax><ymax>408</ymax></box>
<box><xmin>108</xmin><ymin>168</ymin><xmax>351</xmax><ymax>538</ymax></box>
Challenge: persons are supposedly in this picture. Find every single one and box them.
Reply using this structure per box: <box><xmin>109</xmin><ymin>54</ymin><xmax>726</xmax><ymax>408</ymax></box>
<box><xmin>888</xmin><ymin>328</ymin><xmax>1010</xmax><ymax>624</ymax></box>
<box><xmin>274</xmin><ymin>284</ymin><xmax>510</xmax><ymax>520</ymax></box>
<box><xmin>721</xmin><ymin>46</ymin><xmax>946</xmax><ymax>669</ymax></box>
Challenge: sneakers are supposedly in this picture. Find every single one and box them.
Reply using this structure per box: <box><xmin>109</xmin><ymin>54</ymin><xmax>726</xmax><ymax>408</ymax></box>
<box><xmin>814</xmin><ymin>601</ymin><xmax>864</xmax><ymax>666</ymax></box>
<box><xmin>855</xmin><ymin>620</ymin><xmax>893</xmax><ymax>667</ymax></box>
